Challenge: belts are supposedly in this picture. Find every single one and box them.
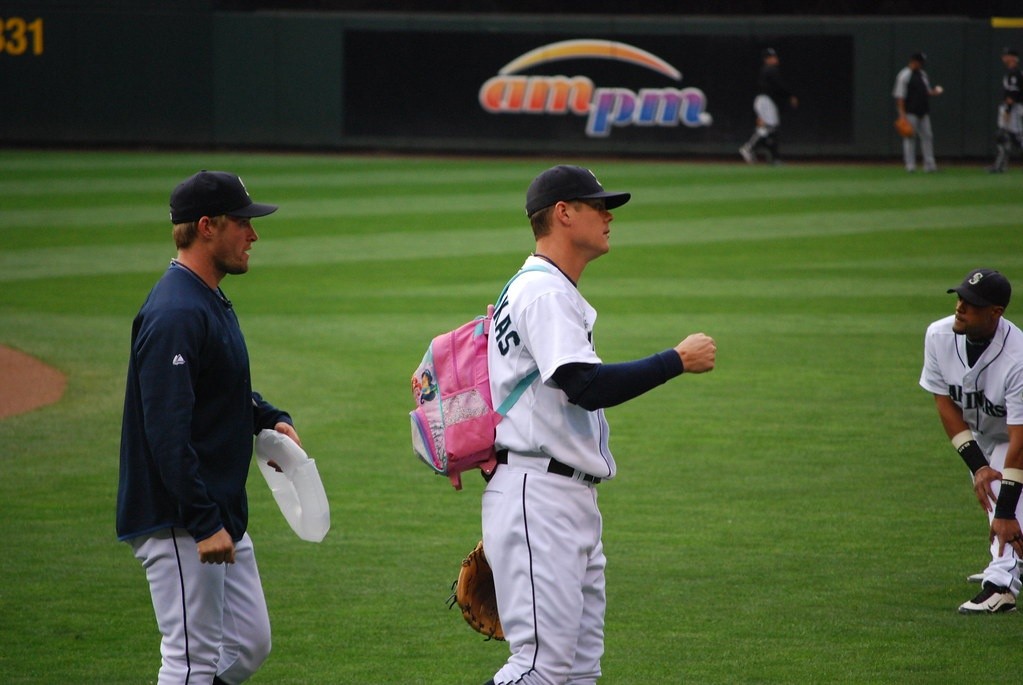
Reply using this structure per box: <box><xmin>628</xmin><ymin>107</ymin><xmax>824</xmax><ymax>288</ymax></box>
<box><xmin>495</xmin><ymin>449</ymin><xmax>600</xmax><ymax>486</ymax></box>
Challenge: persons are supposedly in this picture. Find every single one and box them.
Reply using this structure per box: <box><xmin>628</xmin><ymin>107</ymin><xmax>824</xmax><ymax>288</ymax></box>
<box><xmin>920</xmin><ymin>271</ymin><xmax>1023</xmax><ymax>614</ymax></box>
<box><xmin>738</xmin><ymin>47</ymin><xmax>797</xmax><ymax>164</ymax></box>
<box><xmin>988</xmin><ymin>45</ymin><xmax>1023</xmax><ymax>172</ymax></box>
<box><xmin>115</xmin><ymin>168</ymin><xmax>300</xmax><ymax>685</ymax></box>
<box><xmin>456</xmin><ymin>165</ymin><xmax>716</xmax><ymax>685</ymax></box>
<box><xmin>892</xmin><ymin>51</ymin><xmax>943</xmax><ymax>174</ymax></box>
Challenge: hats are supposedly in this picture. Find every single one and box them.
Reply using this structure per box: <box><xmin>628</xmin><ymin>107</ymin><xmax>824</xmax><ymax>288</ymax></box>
<box><xmin>913</xmin><ymin>52</ymin><xmax>928</xmax><ymax>64</ymax></box>
<box><xmin>526</xmin><ymin>165</ymin><xmax>631</xmax><ymax>219</ymax></box>
<box><xmin>170</xmin><ymin>170</ymin><xmax>278</xmax><ymax>224</ymax></box>
<box><xmin>946</xmin><ymin>268</ymin><xmax>1010</xmax><ymax>309</ymax></box>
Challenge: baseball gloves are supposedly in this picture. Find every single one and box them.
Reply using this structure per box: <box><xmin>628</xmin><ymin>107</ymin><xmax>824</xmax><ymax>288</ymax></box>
<box><xmin>894</xmin><ymin>118</ymin><xmax>913</xmax><ymax>137</ymax></box>
<box><xmin>456</xmin><ymin>537</ymin><xmax>505</xmax><ymax>641</ymax></box>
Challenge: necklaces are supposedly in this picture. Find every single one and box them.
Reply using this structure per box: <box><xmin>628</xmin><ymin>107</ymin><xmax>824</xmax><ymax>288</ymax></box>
<box><xmin>172</xmin><ymin>259</ymin><xmax>233</xmax><ymax>308</ymax></box>
<box><xmin>532</xmin><ymin>254</ymin><xmax>578</xmax><ymax>288</ymax></box>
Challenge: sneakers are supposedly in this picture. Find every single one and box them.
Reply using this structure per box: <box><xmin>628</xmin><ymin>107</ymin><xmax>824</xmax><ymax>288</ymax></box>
<box><xmin>967</xmin><ymin>570</ymin><xmax>1023</xmax><ymax>582</ymax></box>
<box><xmin>957</xmin><ymin>581</ymin><xmax>1017</xmax><ymax>614</ymax></box>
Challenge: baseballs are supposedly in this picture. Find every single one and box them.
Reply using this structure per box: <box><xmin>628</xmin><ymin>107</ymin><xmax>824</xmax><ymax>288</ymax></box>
<box><xmin>936</xmin><ymin>85</ymin><xmax>943</xmax><ymax>93</ymax></box>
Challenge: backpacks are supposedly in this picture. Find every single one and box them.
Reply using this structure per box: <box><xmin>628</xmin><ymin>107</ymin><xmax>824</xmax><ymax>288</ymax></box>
<box><xmin>408</xmin><ymin>266</ymin><xmax>555</xmax><ymax>477</ymax></box>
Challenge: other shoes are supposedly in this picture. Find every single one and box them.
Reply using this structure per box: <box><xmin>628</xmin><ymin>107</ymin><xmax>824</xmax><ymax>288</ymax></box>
<box><xmin>739</xmin><ymin>148</ymin><xmax>753</xmax><ymax>163</ymax></box>
